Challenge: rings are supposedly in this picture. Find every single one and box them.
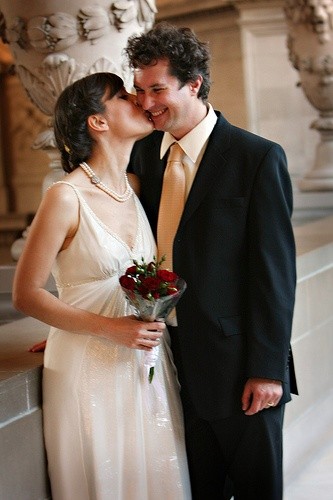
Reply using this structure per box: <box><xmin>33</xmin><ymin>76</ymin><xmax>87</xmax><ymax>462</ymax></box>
<box><xmin>267</xmin><ymin>402</ymin><xmax>274</xmax><ymax>407</ymax></box>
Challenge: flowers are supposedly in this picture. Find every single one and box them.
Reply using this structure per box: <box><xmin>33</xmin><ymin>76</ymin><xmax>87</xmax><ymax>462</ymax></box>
<box><xmin>118</xmin><ymin>254</ymin><xmax>187</xmax><ymax>383</ymax></box>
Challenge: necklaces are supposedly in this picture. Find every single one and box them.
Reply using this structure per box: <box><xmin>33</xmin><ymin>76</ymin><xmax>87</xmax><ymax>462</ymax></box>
<box><xmin>79</xmin><ymin>156</ymin><xmax>136</xmax><ymax>202</ymax></box>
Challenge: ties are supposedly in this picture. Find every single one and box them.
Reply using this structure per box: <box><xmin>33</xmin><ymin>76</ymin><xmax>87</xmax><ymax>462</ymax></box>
<box><xmin>157</xmin><ymin>145</ymin><xmax>187</xmax><ymax>319</ymax></box>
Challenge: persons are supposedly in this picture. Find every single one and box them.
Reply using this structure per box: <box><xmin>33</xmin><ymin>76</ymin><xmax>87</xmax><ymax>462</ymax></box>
<box><xmin>27</xmin><ymin>21</ymin><xmax>299</xmax><ymax>500</ymax></box>
<box><xmin>12</xmin><ymin>71</ymin><xmax>192</xmax><ymax>500</ymax></box>
